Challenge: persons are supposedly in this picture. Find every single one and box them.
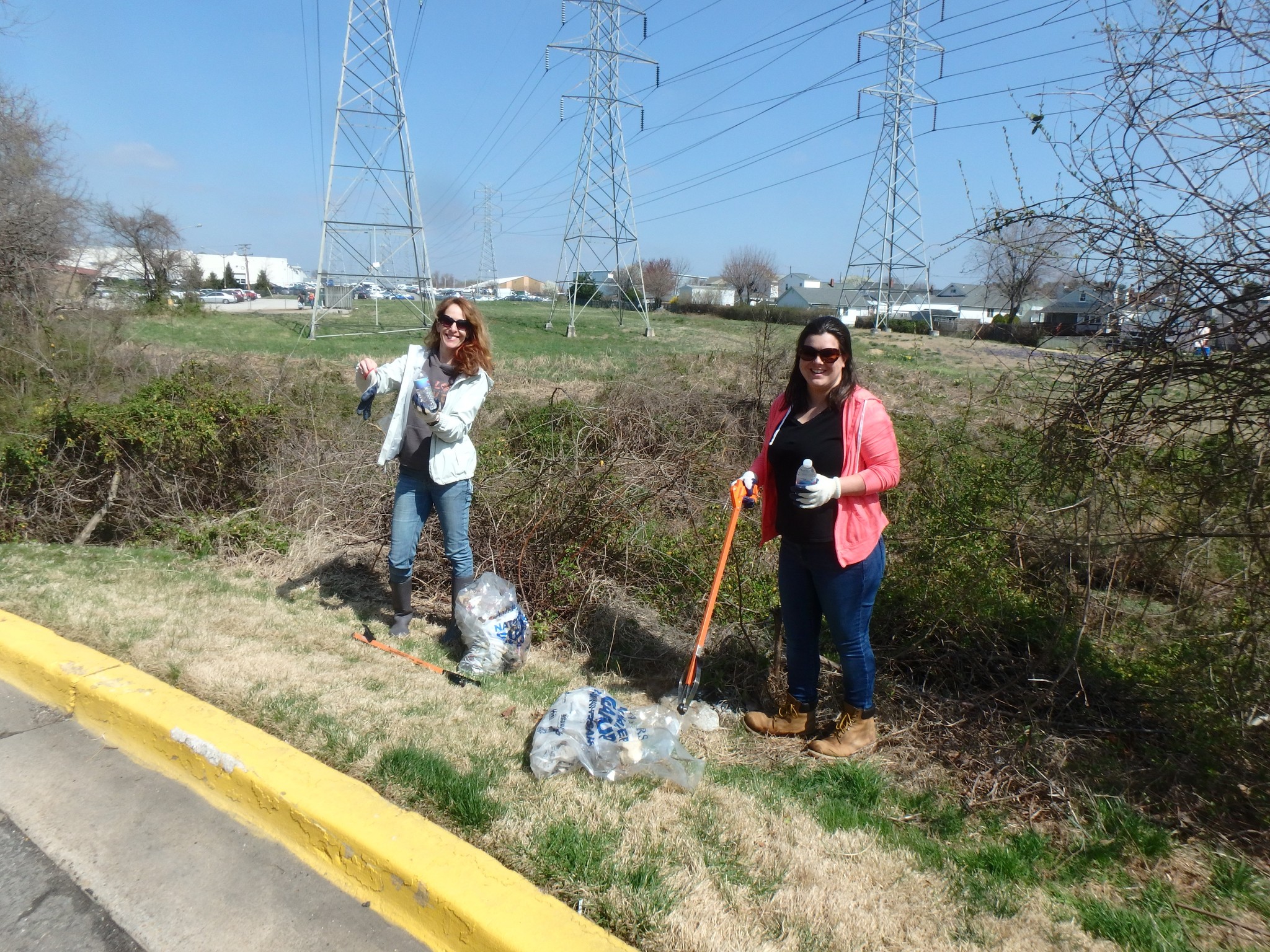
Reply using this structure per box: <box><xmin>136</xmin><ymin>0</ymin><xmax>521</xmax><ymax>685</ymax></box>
<box><xmin>297</xmin><ymin>292</ymin><xmax>304</xmax><ymax>310</ymax></box>
<box><xmin>309</xmin><ymin>292</ymin><xmax>315</xmax><ymax>309</ymax></box>
<box><xmin>731</xmin><ymin>316</ymin><xmax>900</xmax><ymax>762</ymax></box>
<box><xmin>301</xmin><ymin>292</ymin><xmax>306</xmax><ymax>295</ymax></box>
<box><xmin>356</xmin><ymin>297</ymin><xmax>496</xmax><ymax>646</ymax></box>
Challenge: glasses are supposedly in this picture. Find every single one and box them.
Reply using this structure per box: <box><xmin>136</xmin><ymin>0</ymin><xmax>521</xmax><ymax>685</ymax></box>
<box><xmin>797</xmin><ymin>345</ymin><xmax>844</xmax><ymax>363</ymax></box>
<box><xmin>438</xmin><ymin>314</ymin><xmax>472</xmax><ymax>331</ymax></box>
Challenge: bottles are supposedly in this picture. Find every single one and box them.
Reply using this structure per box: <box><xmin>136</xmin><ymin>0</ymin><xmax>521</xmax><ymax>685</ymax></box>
<box><xmin>796</xmin><ymin>461</ymin><xmax>818</xmax><ymax>499</ymax></box>
<box><xmin>411</xmin><ymin>366</ymin><xmax>438</xmax><ymax>411</ymax></box>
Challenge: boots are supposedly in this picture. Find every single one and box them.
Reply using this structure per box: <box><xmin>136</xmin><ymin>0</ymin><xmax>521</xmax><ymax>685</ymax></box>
<box><xmin>439</xmin><ymin>573</ymin><xmax>474</xmax><ymax>645</ymax></box>
<box><xmin>806</xmin><ymin>703</ymin><xmax>877</xmax><ymax>759</ymax></box>
<box><xmin>389</xmin><ymin>576</ymin><xmax>412</xmax><ymax>637</ymax></box>
<box><xmin>741</xmin><ymin>691</ymin><xmax>818</xmax><ymax>738</ymax></box>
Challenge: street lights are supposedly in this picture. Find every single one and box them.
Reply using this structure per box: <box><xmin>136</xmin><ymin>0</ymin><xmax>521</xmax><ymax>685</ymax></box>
<box><xmin>200</xmin><ymin>246</ymin><xmax>227</xmax><ymax>289</ymax></box>
<box><xmin>364</xmin><ymin>231</ymin><xmax>371</xmax><ymax>281</ymax></box>
<box><xmin>166</xmin><ymin>224</ymin><xmax>201</xmax><ymax>277</ymax></box>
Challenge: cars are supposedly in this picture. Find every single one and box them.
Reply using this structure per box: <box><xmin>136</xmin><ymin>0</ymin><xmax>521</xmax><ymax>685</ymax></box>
<box><xmin>270</xmin><ymin>282</ymin><xmax>317</xmax><ymax>295</ymax></box>
<box><xmin>503</xmin><ymin>293</ymin><xmax>557</xmax><ymax>302</ymax></box>
<box><xmin>344</xmin><ymin>285</ymin><xmax>414</xmax><ymax>301</ymax></box>
<box><xmin>398</xmin><ymin>284</ymin><xmax>475</xmax><ymax>300</ymax></box>
<box><xmin>92</xmin><ymin>284</ymin><xmax>261</xmax><ymax>304</ymax></box>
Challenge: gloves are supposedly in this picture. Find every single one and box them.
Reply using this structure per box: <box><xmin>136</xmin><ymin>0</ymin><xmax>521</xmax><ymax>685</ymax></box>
<box><xmin>412</xmin><ymin>393</ymin><xmax>441</xmax><ymax>426</ymax></box>
<box><xmin>357</xmin><ymin>368</ymin><xmax>379</xmax><ymax>420</ymax></box>
<box><xmin>796</xmin><ymin>474</ymin><xmax>841</xmax><ymax>509</ymax></box>
<box><xmin>731</xmin><ymin>470</ymin><xmax>759</xmax><ymax>508</ymax></box>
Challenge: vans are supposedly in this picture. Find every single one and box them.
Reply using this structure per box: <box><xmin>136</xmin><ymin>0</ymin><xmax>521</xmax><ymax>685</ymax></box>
<box><xmin>363</xmin><ymin>281</ymin><xmax>374</xmax><ymax>285</ymax></box>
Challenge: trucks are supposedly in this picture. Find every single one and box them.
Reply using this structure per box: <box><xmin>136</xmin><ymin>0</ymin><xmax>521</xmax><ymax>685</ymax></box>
<box><xmin>325</xmin><ymin>280</ymin><xmax>333</xmax><ymax>286</ymax></box>
<box><xmin>515</xmin><ymin>291</ymin><xmax>530</xmax><ymax>296</ymax></box>
<box><xmin>498</xmin><ymin>289</ymin><xmax>517</xmax><ymax>297</ymax></box>
